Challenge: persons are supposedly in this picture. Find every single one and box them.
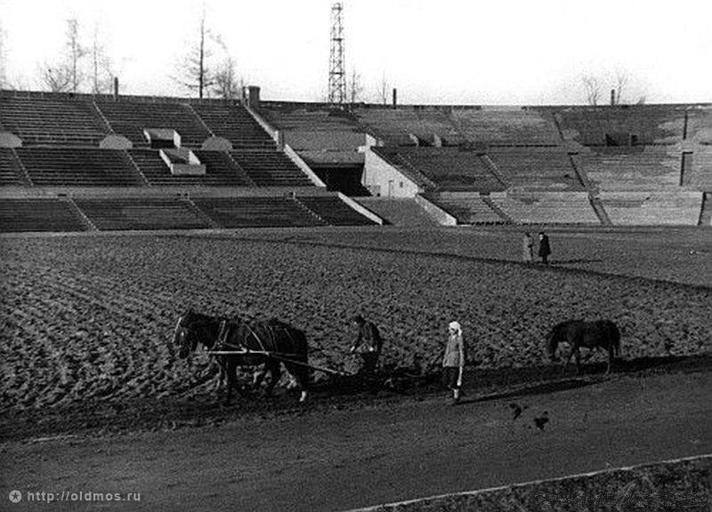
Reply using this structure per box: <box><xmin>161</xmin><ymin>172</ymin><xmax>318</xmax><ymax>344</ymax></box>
<box><xmin>522</xmin><ymin>231</ymin><xmax>534</xmax><ymax>265</ymax></box>
<box><xmin>349</xmin><ymin>315</ymin><xmax>382</xmax><ymax>376</ymax></box>
<box><xmin>537</xmin><ymin>231</ymin><xmax>551</xmax><ymax>264</ymax></box>
<box><xmin>439</xmin><ymin>320</ymin><xmax>465</xmax><ymax>407</ymax></box>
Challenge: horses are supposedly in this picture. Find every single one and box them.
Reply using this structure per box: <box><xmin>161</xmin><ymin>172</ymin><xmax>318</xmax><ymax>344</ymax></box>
<box><xmin>170</xmin><ymin>307</ymin><xmax>293</xmax><ymax>399</ymax></box>
<box><xmin>545</xmin><ymin>318</ymin><xmax>622</xmax><ymax>378</ymax></box>
<box><xmin>177</xmin><ymin>306</ymin><xmax>311</xmax><ymax>402</ymax></box>
